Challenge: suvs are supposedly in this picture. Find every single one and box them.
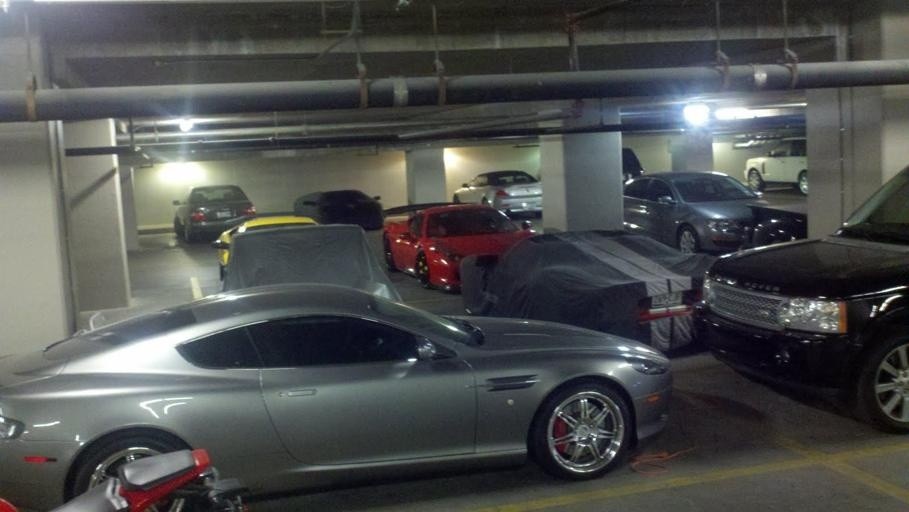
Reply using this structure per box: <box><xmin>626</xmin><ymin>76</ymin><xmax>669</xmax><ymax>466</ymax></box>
<box><xmin>743</xmin><ymin>137</ymin><xmax>809</xmax><ymax>196</ymax></box>
<box><xmin>703</xmin><ymin>163</ymin><xmax>908</xmax><ymax>434</ymax></box>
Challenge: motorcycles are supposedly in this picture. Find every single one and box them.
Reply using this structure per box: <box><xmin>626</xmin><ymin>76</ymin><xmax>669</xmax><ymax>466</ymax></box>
<box><xmin>1</xmin><ymin>450</ymin><xmax>247</xmax><ymax>512</ymax></box>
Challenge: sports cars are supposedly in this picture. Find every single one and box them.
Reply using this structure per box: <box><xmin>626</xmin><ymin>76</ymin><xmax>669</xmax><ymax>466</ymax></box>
<box><xmin>210</xmin><ymin>211</ymin><xmax>322</xmax><ymax>280</ymax></box>
<box><xmin>383</xmin><ymin>205</ymin><xmax>536</xmax><ymax>292</ymax></box>
<box><xmin>460</xmin><ymin>229</ymin><xmax>713</xmax><ymax>352</ymax></box>
<box><xmin>294</xmin><ymin>190</ymin><xmax>384</xmax><ymax>230</ymax></box>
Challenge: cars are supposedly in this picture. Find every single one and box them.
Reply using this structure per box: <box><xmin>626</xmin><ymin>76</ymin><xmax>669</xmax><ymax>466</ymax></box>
<box><xmin>622</xmin><ymin>147</ymin><xmax>645</xmax><ymax>184</ymax></box>
<box><xmin>0</xmin><ymin>282</ymin><xmax>673</xmax><ymax>504</ymax></box>
<box><xmin>173</xmin><ymin>185</ymin><xmax>257</xmax><ymax>241</ymax></box>
<box><xmin>453</xmin><ymin>170</ymin><xmax>543</xmax><ymax>217</ymax></box>
<box><xmin>226</xmin><ymin>224</ymin><xmax>400</xmax><ymax>310</ymax></box>
<box><xmin>624</xmin><ymin>171</ymin><xmax>770</xmax><ymax>253</ymax></box>
